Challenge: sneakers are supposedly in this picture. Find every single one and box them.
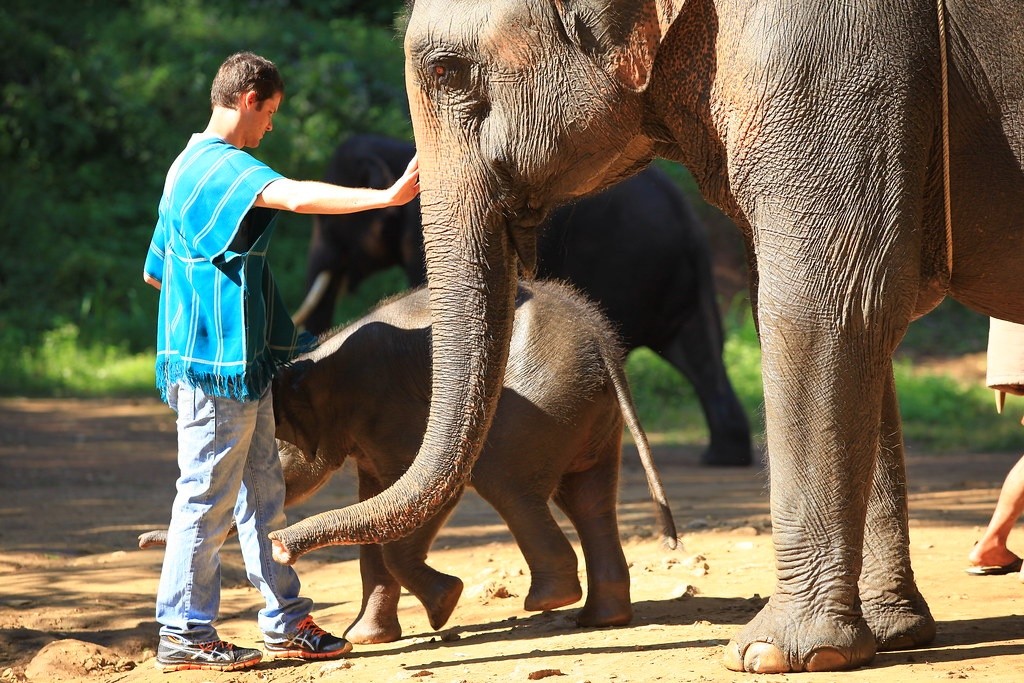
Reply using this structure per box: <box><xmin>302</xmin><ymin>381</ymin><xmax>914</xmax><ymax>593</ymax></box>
<box><xmin>264</xmin><ymin>614</ymin><xmax>353</xmax><ymax>660</ymax></box>
<box><xmin>154</xmin><ymin>633</ymin><xmax>263</xmax><ymax>673</ymax></box>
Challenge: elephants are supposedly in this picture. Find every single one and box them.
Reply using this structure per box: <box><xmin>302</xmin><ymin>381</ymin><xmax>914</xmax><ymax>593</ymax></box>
<box><xmin>141</xmin><ymin>0</ymin><xmax>1024</xmax><ymax>674</ymax></box>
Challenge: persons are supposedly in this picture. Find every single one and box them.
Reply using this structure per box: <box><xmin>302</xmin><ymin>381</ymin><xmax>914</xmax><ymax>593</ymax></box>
<box><xmin>964</xmin><ymin>455</ymin><xmax>1024</xmax><ymax>575</ymax></box>
<box><xmin>142</xmin><ymin>51</ymin><xmax>421</xmax><ymax>672</ymax></box>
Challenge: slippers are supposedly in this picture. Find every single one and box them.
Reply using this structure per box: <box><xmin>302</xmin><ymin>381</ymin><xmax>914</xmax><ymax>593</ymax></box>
<box><xmin>967</xmin><ymin>559</ymin><xmax>1024</xmax><ymax>575</ymax></box>
